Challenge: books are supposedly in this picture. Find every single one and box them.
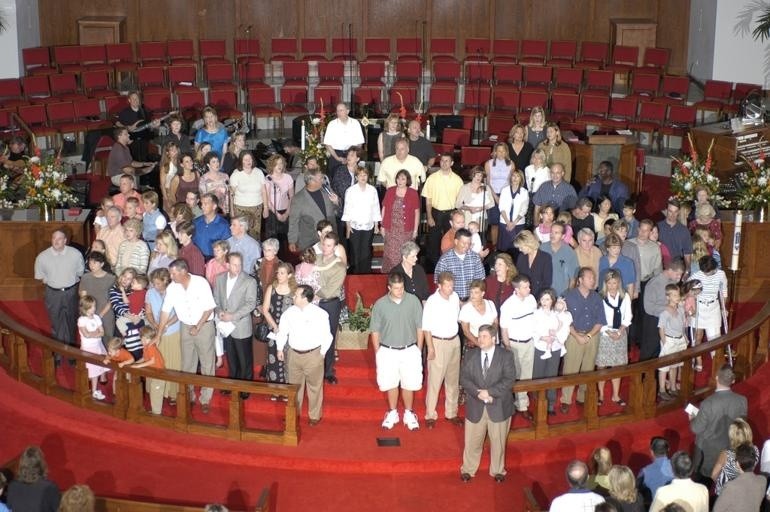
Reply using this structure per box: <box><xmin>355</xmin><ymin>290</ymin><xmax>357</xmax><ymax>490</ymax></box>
<box><xmin>737</xmin><ymin>133</ymin><xmax>770</xmax><ymax>164</ymax></box>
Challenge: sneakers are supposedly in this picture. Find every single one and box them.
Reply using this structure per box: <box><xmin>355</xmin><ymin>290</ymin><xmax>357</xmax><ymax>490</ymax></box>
<box><xmin>381</xmin><ymin>409</ymin><xmax>464</xmax><ymax>430</ymax></box>
<box><xmin>92</xmin><ymin>390</ymin><xmax>105</xmax><ymax>400</ymax></box>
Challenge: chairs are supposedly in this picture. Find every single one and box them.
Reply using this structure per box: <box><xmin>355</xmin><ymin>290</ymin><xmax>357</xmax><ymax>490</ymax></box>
<box><xmin>1</xmin><ymin>33</ymin><xmax>767</xmax><ymax>165</ymax></box>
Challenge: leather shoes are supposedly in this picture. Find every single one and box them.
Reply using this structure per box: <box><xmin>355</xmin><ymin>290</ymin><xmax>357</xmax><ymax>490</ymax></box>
<box><xmin>658</xmin><ymin>390</ymin><xmax>680</xmax><ymax>400</ymax></box>
<box><xmin>326</xmin><ymin>376</ymin><xmax>336</xmax><ymax>384</ymax></box>
<box><xmin>521</xmin><ymin>398</ymin><xmax>626</xmax><ymax>419</ymax></box>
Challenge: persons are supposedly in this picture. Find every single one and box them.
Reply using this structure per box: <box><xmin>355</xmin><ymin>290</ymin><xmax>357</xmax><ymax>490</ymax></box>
<box><xmin>1</xmin><ymin>136</ymin><xmax>28</xmax><ymax>180</ymax></box>
<box><xmin>369</xmin><ymin>104</ymin><xmax>728</xmax><ymax>483</ymax></box>
<box><xmin>34</xmin><ymin>91</ymin><xmax>382</xmax><ymax>426</ymax></box>
<box><xmin>0</xmin><ymin>445</ymin><xmax>229</xmax><ymax>512</ymax></box>
<box><xmin>549</xmin><ymin>363</ymin><xmax>769</xmax><ymax>512</ymax></box>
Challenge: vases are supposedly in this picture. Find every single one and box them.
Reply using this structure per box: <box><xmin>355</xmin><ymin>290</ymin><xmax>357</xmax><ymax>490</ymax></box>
<box><xmin>751</xmin><ymin>202</ymin><xmax>769</xmax><ymax>224</ymax></box>
<box><xmin>36</xmin><ymin>201</ymin><xmax>58</xmax><ymax>222</ymax></box>
<box><xmin>333</xmin><ymin>330</ymin><xmax>371</xmax><ymax>352</ymax></box>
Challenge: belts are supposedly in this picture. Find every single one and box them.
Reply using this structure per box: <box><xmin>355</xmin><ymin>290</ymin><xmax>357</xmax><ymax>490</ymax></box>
<box><xmin>47</xmin><ymin>285</ymin><xmax>78</xmax><ymax>291</ymax></box>
<box><xmin>292</xmin><ymin>346</ymin><xmax>319</xmax><ymax>354</ymax></box>
<box><xmin>509</xmin><ymin>339</ymin><xmax>532</xmax><ymax>343</ymax></box>
<box><xmin>699</xmin><ymin>299</ymin><xmax>718</xmax><ymax>303</ymax></box>
<box><xmin>379</xmin><ymin>343</ymin><xmax>415</xmax><ymax>349</ymax></box>
<box><xmin>432</xmin><ymin>336</ymin><xmax>457</xmax><ymax>340</ymax></box>
<box><xmin>144</xmin><ymin>240</ymin><xmax>154</xmax><ymax>242</ymax></box>
<box><xmin>665</xmin><ymin>334</ymin><xmax>683</xmax><ymax>339</ymax></box>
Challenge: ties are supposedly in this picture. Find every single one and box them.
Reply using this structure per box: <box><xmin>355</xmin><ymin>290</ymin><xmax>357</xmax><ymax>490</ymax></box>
<box><xmin>484</xmin><ymin>353</ymin><xmax>488</xmax><ymax>377</ymax></box>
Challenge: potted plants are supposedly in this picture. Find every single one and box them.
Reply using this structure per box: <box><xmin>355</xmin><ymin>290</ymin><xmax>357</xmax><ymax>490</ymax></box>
<box><xmin>331</xmin><ymin>288</ymin><xmax>375</xmax><ymax>333</ymax></box>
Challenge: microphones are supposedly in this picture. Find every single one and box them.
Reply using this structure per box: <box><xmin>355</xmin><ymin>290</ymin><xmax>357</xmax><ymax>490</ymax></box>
<box><xmin>531</xmin><ymin>177</ymin><xmax>535</xmax><ymax>190</ymax></box>
<box><xmin>225</xmin><ymin>180</ymin><xmax>235</xmax><ymax>195</ymax></box>
<box><xmin>322</xmin><ymin>184</ymin><xmax>332</xmax><ymax>196</ymax></box>
<box><xmin>267</xmin><ymin>176</ymin><xmax>281</xmax><ymax>191</ymax></box>
<box><xmin>483</xmin><ymin>174</ymin><xmax>487</xmax><ymax>191</ymax></box>
<box><xmin>419</xmin><ymin>176</ymin><xmax>422</xmax><ymax>186</ymax></box>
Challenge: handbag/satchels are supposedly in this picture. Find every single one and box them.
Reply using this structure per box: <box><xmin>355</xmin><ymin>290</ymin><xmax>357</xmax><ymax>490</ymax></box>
<box><xmin>254</xmin><ymin>322</ymin><xmax>270</xmax><ymax>342</ymax></box>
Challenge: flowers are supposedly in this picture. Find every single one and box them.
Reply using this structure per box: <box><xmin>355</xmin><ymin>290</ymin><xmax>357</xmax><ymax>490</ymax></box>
<box><xmin>725</xmin><ymin>140</ymin><xmax>769</xmax><ymax>213</ymax></box>
<box><xmin>665</xmin><ymin>131</ymin><xmax>734</xmax><ymax>212</ymax></box>
<box><xmin>1</xmin><ymin>137</ymin><xmax>89</xmax><ymax>216</ymax></box>
